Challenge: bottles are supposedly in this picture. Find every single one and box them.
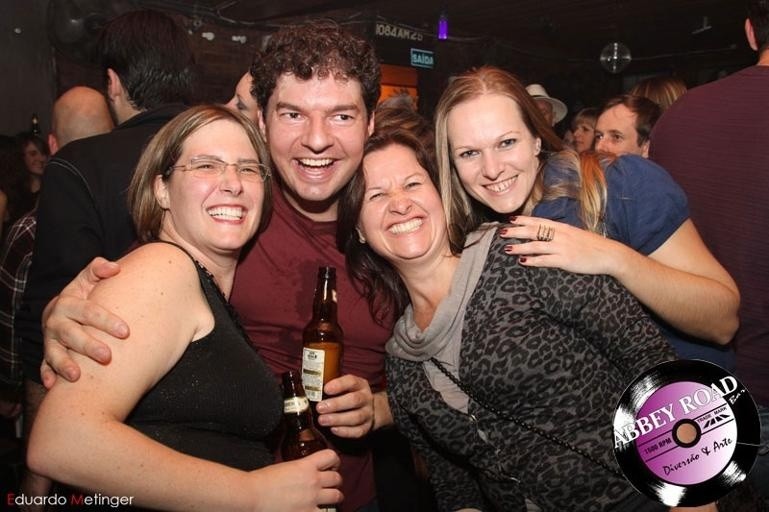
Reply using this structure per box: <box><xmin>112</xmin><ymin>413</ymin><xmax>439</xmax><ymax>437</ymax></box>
<box><xmin>301</xmin><ymin>265</ymin><xmax>345</xmax><ymax>441</ymax></box>
<box><xmin>281</xmin><ymin>369</ymin><xmax>342</xmax><ymax>512</ymax></box>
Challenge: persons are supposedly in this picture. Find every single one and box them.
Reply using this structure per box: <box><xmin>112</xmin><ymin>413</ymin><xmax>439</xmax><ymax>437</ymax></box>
<box><xmin>23</xmin><ymin>104</ymin><xmax>347</xmax><ymax>512</ymax></box>
<box><xmin>373</xmin><ymin>107</ymin><xmax>435</xmax><ymax>163</ymax></box>
<box><xmin>1</xmin><ymin>134</ymin><xmax>26</xmax><ymax>249</ymax></box>
<box><xmin>525</xmin><ymin>76</ymin><xmax>691</xmax><ymax>159</ymax></box>
<box><xmin>433</xmin><ymin>63</ymin><xmax>744</xmax><ymax>377</ymax></box>
<box><xmin>41</xmin><ymin>14</ymin><xmax>396</xmax><ymax>512</ymax></box>
<box><xmin>22</xmin><ymin>6</ymin><xmax>204</xmax><ymax>510</ymax></box>
<box><xmin>13</xmin><ymin>133</ymin><xmax>47</xmax><ymax>218</ymax></box>
<box><xmin>335</xmin><ymin>129</ymin><xmax>719</xmax><ymax>512</ymax></box>
<box><xmin>648</xmin><ymin>0</ymin><xmax>769</xmax><ymax>512</ymax></box>
<box><xmin>0</xmin><ymin>85</ymin><xmax>117</xmax><ymax>423</ymax></box>
<box><xmin>223</xmin><ymin>65</ymin><xmax>261</xmax><ymax>128</ymax></box>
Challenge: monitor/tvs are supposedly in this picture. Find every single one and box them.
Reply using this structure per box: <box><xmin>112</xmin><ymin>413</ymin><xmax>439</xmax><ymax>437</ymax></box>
<box><xmin>379</xmin><ymin>83</ymin><xmax>417</xmax><ymax>111</ymax></box>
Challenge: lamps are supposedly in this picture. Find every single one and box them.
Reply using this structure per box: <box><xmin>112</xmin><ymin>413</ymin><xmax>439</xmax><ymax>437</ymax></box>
<box><xmin>436</xmin><ymin>12</ymin><xmax>450</xmax><ymax>41</ymax></box>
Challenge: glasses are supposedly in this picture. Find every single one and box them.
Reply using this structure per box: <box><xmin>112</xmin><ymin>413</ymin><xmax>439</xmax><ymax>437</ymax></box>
<box><xmin>162</xmin><ymin>156</ymin><xmax>273</xmax><ymax>184</ymax></box>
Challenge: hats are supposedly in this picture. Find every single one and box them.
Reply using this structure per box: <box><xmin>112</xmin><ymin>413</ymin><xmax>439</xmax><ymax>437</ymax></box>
<box><xmin>522</xmin><ymin>82</ymin><xmax>570</xmax><ymax>131</ymax></box>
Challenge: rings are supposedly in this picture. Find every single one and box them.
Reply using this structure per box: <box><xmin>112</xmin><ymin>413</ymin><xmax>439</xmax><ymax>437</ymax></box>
<box><xmin>536</xmin><ymin>224</ymin><xmax>556</xmax><ymax>241</ymax></box>
